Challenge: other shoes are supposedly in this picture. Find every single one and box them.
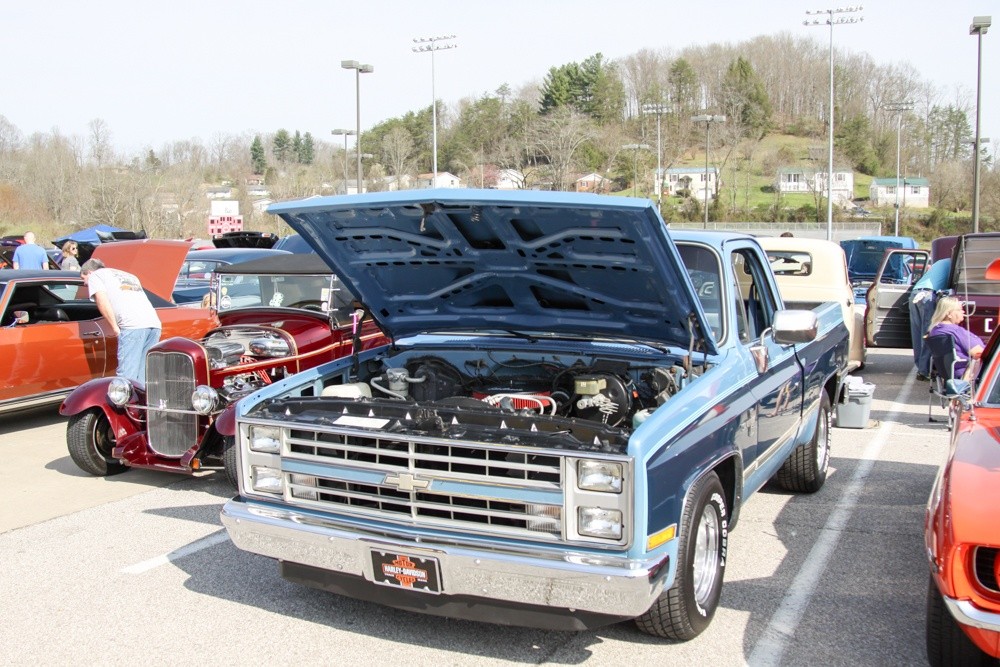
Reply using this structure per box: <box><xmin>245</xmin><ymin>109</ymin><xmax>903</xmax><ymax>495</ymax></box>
<box><xmin>917</xmin><ymin>370</ymin><xmax>936</xmax><ymax>381</ymax></box>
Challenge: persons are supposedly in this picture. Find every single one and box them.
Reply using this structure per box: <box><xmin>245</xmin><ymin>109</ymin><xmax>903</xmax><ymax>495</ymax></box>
<box><xmin>927</xmin><ymin>296</ymin><xmax>987</xmax><ymax>381</ymax></box>
<box><xmin>61</xmin><ymin>240</ymin><xmax>81</xmax><ymax>271</ymax></box>
<box><xmin>909</xmin><ymin>257</ymin><xmax>953</xmax><ymax>380</ymax></box>
<box><xmin>11</xmin><ymin>232</ymin><xmax>49</xmax><ymax>271</ymax></box>
<box><xmin>79</xmin><ymin>259</ymin><xmax>162</xmax><ymax>389</ymax></box>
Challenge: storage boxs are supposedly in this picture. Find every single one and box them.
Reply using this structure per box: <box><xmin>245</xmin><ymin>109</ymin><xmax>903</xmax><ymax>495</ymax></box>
<box><xmin>836</xmin><ymin>382</ymin><xmax>876</xmax><ymax>428</ymax></box>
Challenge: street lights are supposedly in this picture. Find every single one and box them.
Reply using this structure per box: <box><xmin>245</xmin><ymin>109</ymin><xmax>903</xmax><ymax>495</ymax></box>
<box><xmin>412</xmin><ymin>32</ymin><xmax>458</xmax><ymax>187</ymax></box>
<box><xmin>622</xmin><ymin>144</ymin><xmax>651</xmax><ymax>197</ymax></box>
<box><xmin>882</xmin><ymin>97</ymin><xmax>914</xmax><ymax>235</ymax></box>
<box><xmin>692</xmin><ymin>114</ymin><xmax>726</xmax><ymax>229</ymax></box>
<box><xmin>802</xmin><ymin>5</ymin><xmax>865</xmax><ymax>240</ymax></box>
<box><xmin>331</xmin><ymin>129</ymin><xmax>357</xmax><ymax>194</ymax></box>
<box><xmin>969</xmin><ymin>15</ymin><xmax>992</xmax><ymax>235</ymax></box>
<box><xmin>341</xmin><ymin>59</ymin><xmax>374</xmax><ymax>194</ymax></box>
<box><xmin>642</xmin><ymin>103</ymin><xmax>678</xmax><ymax>214</ymax></box>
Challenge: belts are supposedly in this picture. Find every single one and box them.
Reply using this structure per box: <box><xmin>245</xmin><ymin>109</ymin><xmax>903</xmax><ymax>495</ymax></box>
<box><xmin>914</xmin><ymin>288</ymin><xmax>936</xmax><ymax>293</ymax></box>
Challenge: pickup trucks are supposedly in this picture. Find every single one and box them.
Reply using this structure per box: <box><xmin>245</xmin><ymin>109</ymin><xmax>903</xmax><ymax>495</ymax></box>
<box><xmin>218</xmin><ymin>184</ymin><xmax>848</xmax><ymax>640</ymax></box>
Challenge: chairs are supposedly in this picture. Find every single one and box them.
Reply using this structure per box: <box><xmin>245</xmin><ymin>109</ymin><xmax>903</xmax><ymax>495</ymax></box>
<box><xmin>923</xmin><ymin>331</ymin><xmax>972</xmax><ymax>430</ymax></box>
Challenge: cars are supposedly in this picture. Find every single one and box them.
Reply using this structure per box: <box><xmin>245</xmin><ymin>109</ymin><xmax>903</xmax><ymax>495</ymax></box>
<box><xmin>925</xmin><ymin>258</ymin><xmax>1000</xmax><ymax>667</ymax></box>
<box><xmin>172</xmin><ymin>230</ymin><xmax>317</xmax><ymax>307</ymax></box>
<box><xmin>0</xmin><ymin>237</ymin><xmax>224</xmax><ymax>420</ymax></box>
<box><xmin>731</xmin><ymin>233</ymin><xmax>866</xmax><ymax>374</ymax></box>
<box><xmin>0</xmin><ymin>235</ymin><xmax>65</xmax><ymax>270</ymax></box>
<box><xmin>58</xmin><ymin>253</ymin><xmax>390</xmax><ymax>491</ymax></box>
<box><xmin>842</xmin><ymin>235</ymin><xmax>920</xmax><ymax>304</ymax></box>
<box><xmin>862</xmin><ymin>232</ymin><xmax>999</xmax><ymax>348</ymax></box>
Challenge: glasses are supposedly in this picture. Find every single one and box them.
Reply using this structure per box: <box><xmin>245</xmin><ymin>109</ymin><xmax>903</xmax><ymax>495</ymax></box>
<box><xmin>70</xmin><ymin>247</ymin><xmax>78</xmax><ymax>250</ymax></box>
